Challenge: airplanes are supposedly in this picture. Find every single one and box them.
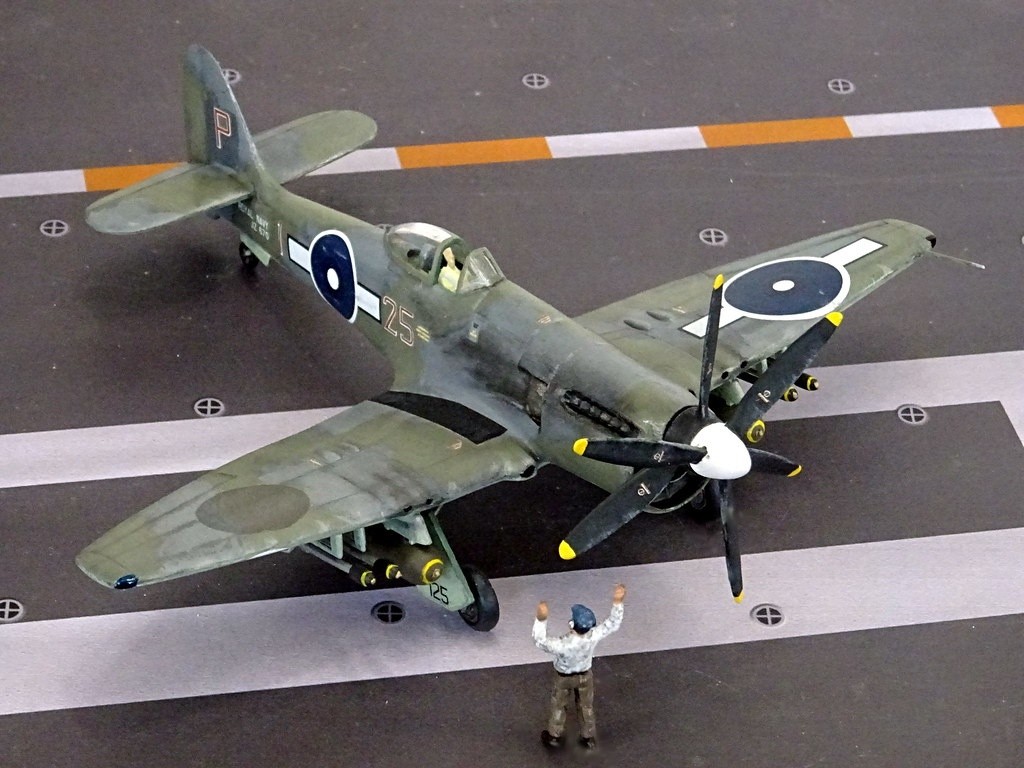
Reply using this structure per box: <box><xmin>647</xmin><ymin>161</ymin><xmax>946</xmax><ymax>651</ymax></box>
<box><xmin>73</xmin><ymin>44</ymin><xmax>938</xmax><ymax>635</ymax></box>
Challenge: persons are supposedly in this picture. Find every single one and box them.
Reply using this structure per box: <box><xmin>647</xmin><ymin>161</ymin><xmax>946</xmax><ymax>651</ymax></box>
<box><xmin>532</xmin><ymin>584</ymin><xmax>626</xmax><ymax>749</ymax></box>
